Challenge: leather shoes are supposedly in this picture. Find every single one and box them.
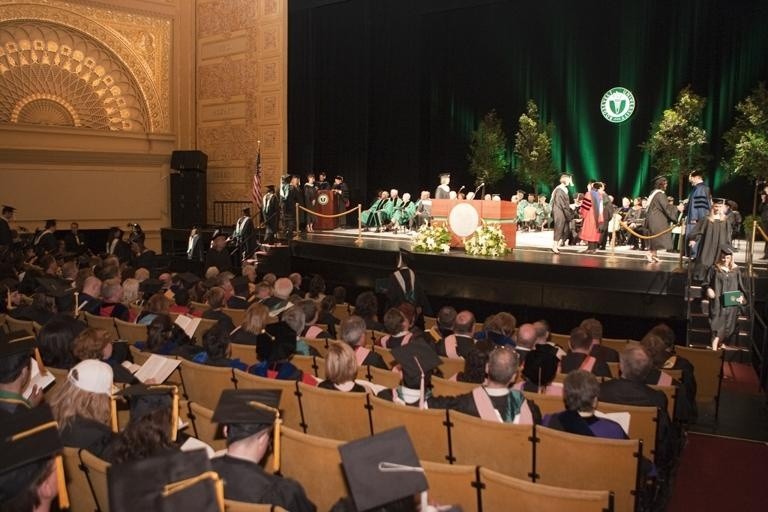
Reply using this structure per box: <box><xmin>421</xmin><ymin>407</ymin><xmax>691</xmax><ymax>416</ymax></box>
<box><xmin>653</xmin><ymin>256</ymin><xmax>660</xmax><ymax>262</ymax></box>
<box><xmin>551</xmin><ymin>238</ymin><xmax>650</xmax><ymax>256</ymax></box>
<box><xmin>644</xmin><ymin>254</ymin><xmax>653</xmax><ymax>263</ymax></box>
<box><xmin>362</xmin><ymin>224</ymin><xmax>419</xmax><ymax>236</ymax></box>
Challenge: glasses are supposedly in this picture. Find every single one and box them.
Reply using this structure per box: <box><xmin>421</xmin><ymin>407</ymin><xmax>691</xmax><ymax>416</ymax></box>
<box><xmin>493</xmin><ymin>345</ymin><xmax>520</xmax><ymax>361</ymax></box>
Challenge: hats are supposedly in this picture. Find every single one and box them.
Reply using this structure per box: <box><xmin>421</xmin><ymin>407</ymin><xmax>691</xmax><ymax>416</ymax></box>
<box><xmin>0</xmin><ymin>329</ymin><xmax>46</xmax><ymax>377</ymax></box>
<box><xmin>105</xmin><ymin>448</ymin><xmax>225</xmax><ymax>512</ymax></box>
<box><xmin>192</xmin><ymin>224</ymin><xmax>200</xmax><ymax>229</ymax></box>
<box><xmin>210</xmin><ymin>389</ymin><xmax>281</xmax><ymax>473</ymax></box>
<box><xmin>389</xmin><ymin>337</ymin><xmax>443</xmax><ymax>410</ymax></box>
<box><xmin>396</xmin><ymin>248</ymin><xmax>413</xmax><ymax>268</ymax></box>
<box><xmin>112</xmin><ymin>381</ymin><xmax>181</xmax><ymax>443</ymax></box>
<box><xmin>524</xmin><ymin>349</ymin><xmax>559</xmax><ymax>394</ymax></box>
<box><xmin>138</xmin><ymin>272</ymin><xmax>251</xmax><ymax>292</ymax></box>
<box><xmin>439</xmin><ymin>172</ymin><xmax>450</xmax><ymax>178</ymax></box>
<box><xmin>2</xmin><ymin>204</ymin><xmax>80</xmax><ymax>317</ymax></box>
<box><xmin>558</xmin><ymin>172</ymin><xmax>574</xmax><ymax>187</ymax></box>
<box><xmin>255</xmin><ymin>321</ymin><xmax>296</xmax><ymax>361</ymax></box>
<box><xmin>1</xmin><ymin>402</ymin><xmax>70</xmax><ymax>510</ymax></box>
<box><xmin>719</xmin><ymin>244</ymin><xmax>739</xmax><ymax>271</ymax></box>
<box><xmin>67</xmin><ymin>359</ymin><xmax>121</xmax><ymax>395</ymax></box>
<box><xmin>651</xmin><ymin>173</ymin><xmax>667</xmax><ymax>182</ymax></box>
<box><xmin>337</xmin><ymin>425</ymin><xmax>428</xmax><ymax>512</ymax></box>
<box><xmin>712</xmin><ymin>198</ymin><xmax>727</xmax><ymax>222</ymax></box>
<box><xmin>241</xmin><ymin>175</ymin><xmax>292</xmax><ymax>216</ymax></box>
<box><xmin>109</xmin><ymin>227</ymin><xmax>119</xmax><ymax>232</ymax></box>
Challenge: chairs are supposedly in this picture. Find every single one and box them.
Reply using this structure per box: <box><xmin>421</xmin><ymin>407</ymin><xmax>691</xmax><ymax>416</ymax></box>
<box><xmin>2</xmin><ymin>263</ymin><xmax>726</xmax><ymax>512</ymax></box>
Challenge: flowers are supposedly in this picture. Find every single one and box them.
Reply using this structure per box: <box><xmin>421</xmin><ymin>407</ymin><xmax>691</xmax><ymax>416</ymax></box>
<box><xmin>462</xmin><ymin>218</ymin><xmax>514</xmax><ymax>258</ymax></box>
<box><xmin>408</xmin><ymin>216</ymin><xmax>455</xmax><ymax>256</ymax></box>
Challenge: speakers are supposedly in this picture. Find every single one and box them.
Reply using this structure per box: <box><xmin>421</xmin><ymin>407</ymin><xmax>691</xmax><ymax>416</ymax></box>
<box><xmin>171</xmin><ymin>150</ymin><xmax>208</xmax><ymax>171</ymax></box>
<box><xmin>170</xmin><ymin>172</ymin><xmax>207</xmax><ymax>227</ymax></box>
